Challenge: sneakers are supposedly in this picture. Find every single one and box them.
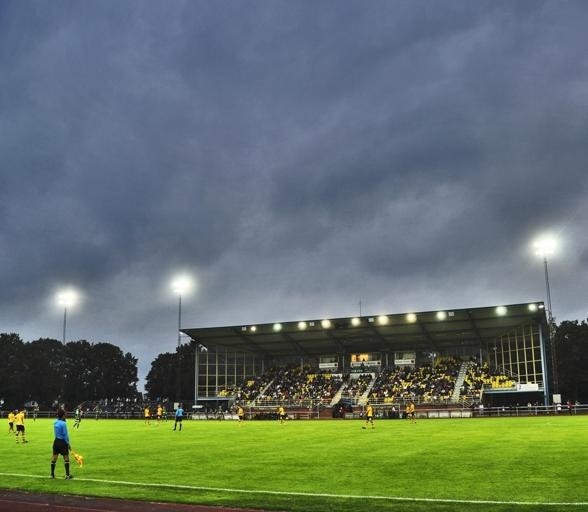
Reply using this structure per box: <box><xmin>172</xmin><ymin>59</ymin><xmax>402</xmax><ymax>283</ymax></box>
<box><xmin>65</xmin><ymin>475</ymin><xmax>73</xmax><ymax>480</ymax></box>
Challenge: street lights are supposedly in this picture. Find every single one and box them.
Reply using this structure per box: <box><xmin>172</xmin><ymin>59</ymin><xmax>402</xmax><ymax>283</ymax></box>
<box><xmin>532</xmin><ymin>236</ymin><xmax>560</xmax><ymax>394</ymax></box>
<box><xmin>59</xmin><ymin>292</ymin><xmax>73</xmax><ymax>343</ymax></box>
<box><xmin>173</xmin><ymin>280</ymin><xmax>187</xmax><ymax>402</ymax></box>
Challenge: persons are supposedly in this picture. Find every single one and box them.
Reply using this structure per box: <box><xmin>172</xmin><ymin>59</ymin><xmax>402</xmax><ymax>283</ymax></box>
<box><xmin>49</xmin><ymin>409</ymin><xmax>75</xmax><ymax>480</ymax></box>
<box><xmin>14</xmin><ymin>408</ymin><xmax>27</xmax><ymax>446</ymax></box>
